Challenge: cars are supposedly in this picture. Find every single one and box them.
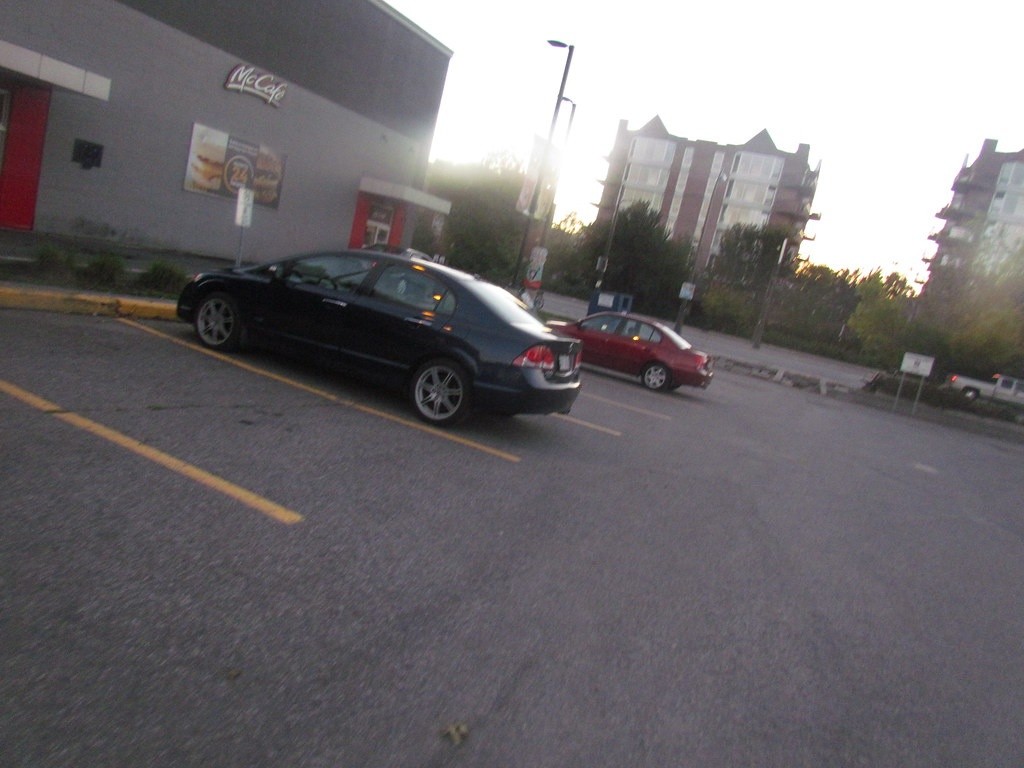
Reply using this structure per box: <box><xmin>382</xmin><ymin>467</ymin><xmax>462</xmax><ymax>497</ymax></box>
<box><xmin>175</xmin><ymin>249</ymin><xmax>584</xmax><ymax>430</ymax></box>
<box><xmin>545</xmin><ymin>311</ymin><xmax>715</xmax><ymax>394</ymax></box>
<box><xmin>361</xmin><ymin>240</ymin><xmax>434</xmax><ymax>296</ymax></box>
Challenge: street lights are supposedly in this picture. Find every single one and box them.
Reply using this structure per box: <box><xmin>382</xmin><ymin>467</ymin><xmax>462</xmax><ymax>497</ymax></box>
<box><xmin>508</xmin><ymin>38</ymin><xmax>575</xmax><ymax>290</ymax></box>
<box><xmin>540</xmin><ymin>96</ymin><xmax>576</xmax><ymax>249</ymax></box>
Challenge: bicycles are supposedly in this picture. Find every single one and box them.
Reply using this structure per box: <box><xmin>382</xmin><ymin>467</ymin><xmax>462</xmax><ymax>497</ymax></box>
<box><xmin>517</xmin><ymin>287</ymin><xmax>545</xmax><ymax>310</ymax></box>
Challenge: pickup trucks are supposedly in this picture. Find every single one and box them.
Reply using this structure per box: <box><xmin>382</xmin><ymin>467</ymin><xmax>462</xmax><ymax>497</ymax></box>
<box><xmin>943</xmin><ymin>373</ymin><xmax>1024</xmax><ymax>408</ymax></box>
